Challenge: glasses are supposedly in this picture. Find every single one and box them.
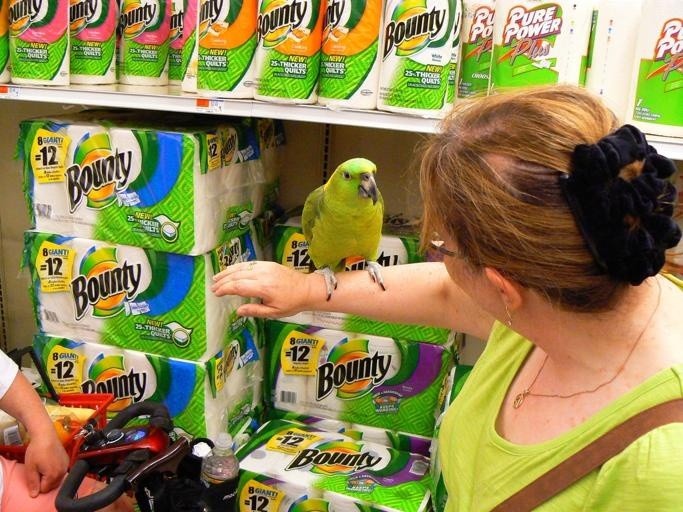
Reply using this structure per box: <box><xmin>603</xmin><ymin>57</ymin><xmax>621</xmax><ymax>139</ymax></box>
<box><xmin>426</xmin><ymin>227</ymin><xmax>455</xmax><ymax>258</ymax></box>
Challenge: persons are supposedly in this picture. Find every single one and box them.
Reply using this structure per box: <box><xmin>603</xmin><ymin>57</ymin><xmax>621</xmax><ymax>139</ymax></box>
<box><xmin>210</xmin><ymin>81</ymin><xmax>683</xmax><ymax>512</ymax></box>
<box><xmin>0</xmin><ymin>349</ymin><xmax>138</xmax><ymax>512</ymax></box>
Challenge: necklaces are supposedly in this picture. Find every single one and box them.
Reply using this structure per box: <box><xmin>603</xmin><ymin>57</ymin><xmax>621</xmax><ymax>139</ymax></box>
<box><xmin>511</xmin><ymin>275</ymin><xmax>662</xmax><ymax>409</ymax></box>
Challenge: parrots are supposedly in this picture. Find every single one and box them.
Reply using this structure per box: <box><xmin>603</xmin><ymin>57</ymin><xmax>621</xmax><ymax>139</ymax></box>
<box><xmin>301</xmin><ymin>157</ymin><xmax>386</xmax><ymax>302</ymax></box>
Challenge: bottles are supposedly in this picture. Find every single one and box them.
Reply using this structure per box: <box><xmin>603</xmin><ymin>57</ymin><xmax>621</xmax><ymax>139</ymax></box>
<box><xmin>199</xmin><ymin>432</ymin><xmax>240</xmax><ymax>512</ymax></box>
